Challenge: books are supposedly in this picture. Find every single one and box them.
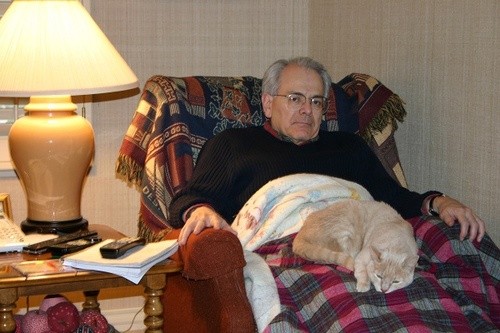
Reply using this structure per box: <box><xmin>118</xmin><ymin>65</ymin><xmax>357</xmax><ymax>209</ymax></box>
<box><xmin>63</xmin><ymin>238</ymin><xmax>179</xmax><ymax>284</ymax></box>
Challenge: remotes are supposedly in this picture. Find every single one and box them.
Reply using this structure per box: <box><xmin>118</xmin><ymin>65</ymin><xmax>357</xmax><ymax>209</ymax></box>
<box><xmin>22</xmin><ymin>231</ymin><xmax>98</xmax><ymax>254</ymax></box>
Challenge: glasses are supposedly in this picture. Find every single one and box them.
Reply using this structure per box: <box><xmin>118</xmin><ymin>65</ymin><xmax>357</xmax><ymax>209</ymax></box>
<box><xmin>269</xmin><ymin>92</ymin><xmax>329</xmax><ymax>111</ymax></box>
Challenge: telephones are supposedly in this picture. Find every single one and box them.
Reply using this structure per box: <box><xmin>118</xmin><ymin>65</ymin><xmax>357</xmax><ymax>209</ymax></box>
<box><xmin>0</xmin><ymin>213</ymin><xmax>30</xmax><ymax>253</ymax></box>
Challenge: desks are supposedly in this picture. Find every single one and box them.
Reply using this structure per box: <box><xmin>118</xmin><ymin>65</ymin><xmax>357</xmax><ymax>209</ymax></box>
<box><xmin>0</xmin><ymin>224</ymin><xmax>188</xmax><ymax>333</ymax></box>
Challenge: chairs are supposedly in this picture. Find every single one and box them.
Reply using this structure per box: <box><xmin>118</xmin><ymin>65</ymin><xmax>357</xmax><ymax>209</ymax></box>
<box><xmin>115</xmin><ymin>74</ymin><xmax>500</xmax><ymax>333</ymax></box>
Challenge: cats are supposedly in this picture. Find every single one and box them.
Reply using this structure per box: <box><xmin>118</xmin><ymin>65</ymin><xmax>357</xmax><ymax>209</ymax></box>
<box><xmin>291</xmin><ymin>198</ymin><xmax>420</xmax><ymax>294</ymax></box>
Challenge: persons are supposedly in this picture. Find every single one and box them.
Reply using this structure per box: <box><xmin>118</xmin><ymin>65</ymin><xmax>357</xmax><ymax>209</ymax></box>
<box><xmin>170</xmin><ymin>57</ymin><xmax>485</xmax><ymax>246</ymax></box>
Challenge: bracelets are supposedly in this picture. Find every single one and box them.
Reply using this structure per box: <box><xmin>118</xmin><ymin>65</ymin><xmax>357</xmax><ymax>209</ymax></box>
<box><xmin>429</xmin><ymin>197</ymin><xmax>439</xmax><ymax>216</ymax></box>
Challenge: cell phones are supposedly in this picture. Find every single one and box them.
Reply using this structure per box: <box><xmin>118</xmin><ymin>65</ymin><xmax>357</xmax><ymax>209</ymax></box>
<box><xmin>99</xmin><ymin>237</ymin><xmax>146</xmax><ymax>260</ymax></box>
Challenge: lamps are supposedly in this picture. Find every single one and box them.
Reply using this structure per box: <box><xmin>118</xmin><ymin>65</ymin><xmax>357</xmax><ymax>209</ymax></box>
<box><xmin>0</xmin><ymin>0</ymin><xmax>139</xmax><ymax>234</ymax></box>
